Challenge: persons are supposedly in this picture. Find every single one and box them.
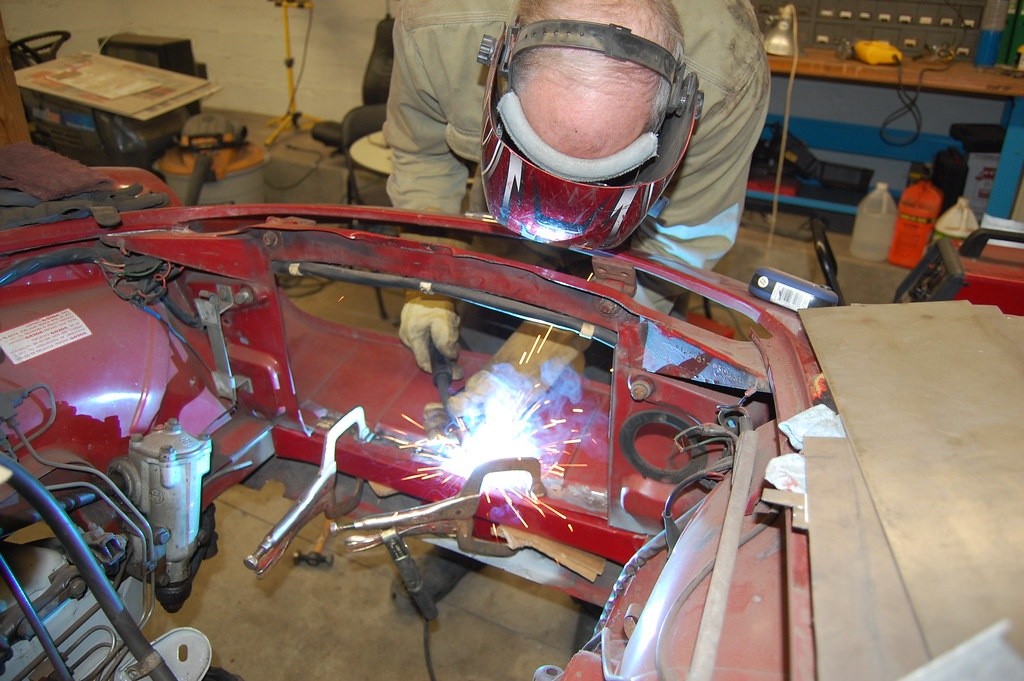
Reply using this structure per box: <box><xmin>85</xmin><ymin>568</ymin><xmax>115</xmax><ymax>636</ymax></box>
<box><xmin>382</xmin><ymin>0</ymin><xmax>772</xmax><ymax>656</ymax></box>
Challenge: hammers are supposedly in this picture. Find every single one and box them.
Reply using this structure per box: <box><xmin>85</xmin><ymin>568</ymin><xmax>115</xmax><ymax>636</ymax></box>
<box><xmin>293</xmin><ymin>519</ymin><xmax>335</xmax><ymax>567</ymax></box>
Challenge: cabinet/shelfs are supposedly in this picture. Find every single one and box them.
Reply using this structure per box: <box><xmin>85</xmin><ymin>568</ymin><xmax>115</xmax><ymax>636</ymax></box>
<box><xmin>746</xmin><ymin>46</ymin><xmax>1024</xmax><ymax>219</ymax></box>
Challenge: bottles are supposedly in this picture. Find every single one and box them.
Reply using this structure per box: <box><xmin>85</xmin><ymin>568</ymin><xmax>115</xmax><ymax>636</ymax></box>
<box><xmin>931</xmin><ymin>197</ymin><xmax>979</xmax><ymax>252</ymax></box>
<box><xmin>849</xmin><ymin>182</ymin><xmax>896</xmax><ymax>263</ymax></box>
<box><xmin>889</xmin><ymin>173</ymin><xmax>942</xmax><ymax>270</ymax></box>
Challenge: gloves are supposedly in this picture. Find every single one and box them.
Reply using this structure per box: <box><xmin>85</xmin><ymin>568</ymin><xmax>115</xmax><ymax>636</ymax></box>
<box><xmin>423</xmin><ymin>371</ymin><xmax>521</xmax><ymax>440</ymax></box>
<box><xmin>399</xmin><ymin>287</ymin><xmax>464</xmax><ymax>379</ymax></box>
